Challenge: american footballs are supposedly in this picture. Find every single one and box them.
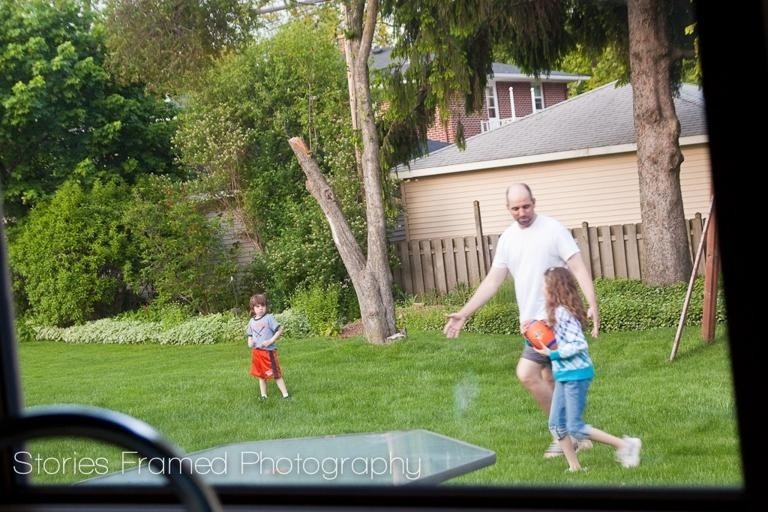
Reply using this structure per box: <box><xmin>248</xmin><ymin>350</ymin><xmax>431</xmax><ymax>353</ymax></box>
<box><xmin>523</xmin><ymin>318</ymin><xmax>556</xmax><ymax>350</ymax></box>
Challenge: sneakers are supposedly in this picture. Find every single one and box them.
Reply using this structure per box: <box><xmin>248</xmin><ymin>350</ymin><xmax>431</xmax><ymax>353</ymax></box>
<box><xmin>617</xmin><ymin>437</ymin><xmax>642</xmax><ymax>468</ymax></box>
<box><xmin>544</xmin><ymin>434</ymin><xmax>593</xmax><ymax>458</ymax></box>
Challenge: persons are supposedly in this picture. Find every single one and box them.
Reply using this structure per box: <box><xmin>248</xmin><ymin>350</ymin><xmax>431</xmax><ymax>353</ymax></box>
<box><xmin>445</xmin><ymin>182</ymin><xmax>642</xmax><ymax>471</ymax></box>
<box><xmin>247</xmin><ymin>295</ymin><xmax>288</xmax><ymax>399</ymax></box>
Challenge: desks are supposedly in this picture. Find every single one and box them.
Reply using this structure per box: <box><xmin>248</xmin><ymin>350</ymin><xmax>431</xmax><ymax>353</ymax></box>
<box><xmin>70</xmin><ymin>429</ymin><xmax>495</xmax><ymax>487</ymax></box>
